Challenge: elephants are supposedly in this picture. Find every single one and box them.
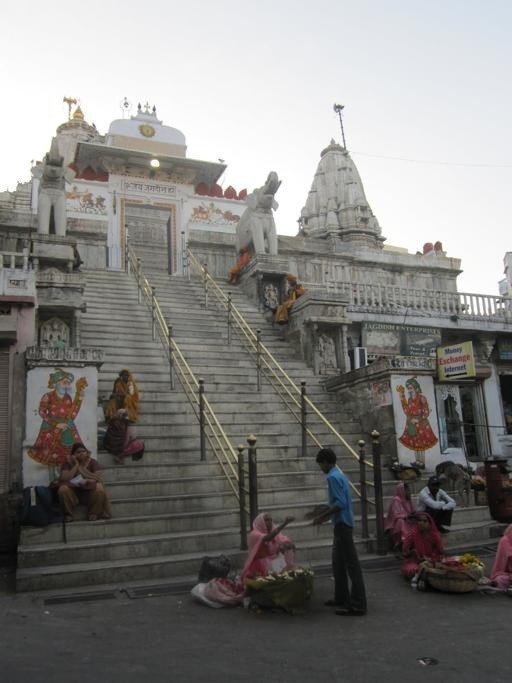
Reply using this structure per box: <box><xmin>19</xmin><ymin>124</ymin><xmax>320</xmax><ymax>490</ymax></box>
<box><xmin>234</xmin><ymin>169</ymin><xmax>283</xmax><ymax>268</ymax></box>
<box><xmin>29</xmin><ymin>136</ymin><xmax>78</xmax><ymax>237</ymax></box>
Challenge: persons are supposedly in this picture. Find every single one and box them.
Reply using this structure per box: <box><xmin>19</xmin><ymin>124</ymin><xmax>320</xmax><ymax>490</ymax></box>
<box><xmin>225</xmin><ymin>245</ymin><xmax>250</xmax><ymax>286</ymax></box>
<box><xmin>415</xmin><ymin>242</ymin><xmax>436</xmax><ymax>257</ymax></box>
<box><xmin>399</xmin><ymin>510</ymin><xmax>446</xmax><ymax>582</ymax></box>
<box><xmin>300</xmin><ymin>446</ymin><xmax>369</xmax><ymax>617</ymax></box>
<box><xmin>102</xmin><ymin>407</ymin><xmax>144</xmax><ymax>465</ymax></box>
<box><xmin>103</xmin><ymin>367</ymin><xmax>140</xmax><ymax>425</ymax></box>
<box><xmin>45</xmin><ymin>441</ymin><xmax>115</xmax><ymax>520</ymax></box>
<box><xmin>396</xmin><ymin>375</ymin><xmax>439</xmax><ymax>470</ymax></box>
<box><xmin>382</xmin><ymin>480</ymin><xmax>415</xmax><ymax>561</ymax></box>
<box><xmin>489</xmin><ymin>522</ymin><xmax>512</xmax><ymax>595</ymax></box>
<box><xmin>415</xmin><ymin>475</ymin><xmax>456</xmax><ymax>534</ymax></box>
<box><xmin>273</xmin><ymin>274</ymin><xmax>306</xmax><ymax>325</ymax></box>
<box><xmin>433</xmin><ymin>240</ymin><xmax>448</xmax><ymax>257</ymax></box>
<box><xmin>23</xmin><ymin>367</ymin><xmax>86</xmax><ymax>484</ymax></box>
<box><xmin>236</xmin><ymin>510</ymin><xmax>299</xmax><ymax>593</ymax></box>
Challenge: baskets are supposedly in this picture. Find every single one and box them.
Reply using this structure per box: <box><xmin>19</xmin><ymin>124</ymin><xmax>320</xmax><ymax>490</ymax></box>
<box><xmin>422</xmin><ymin>564</ymin><xmax>477</xmax><ymax>593</ymax></box>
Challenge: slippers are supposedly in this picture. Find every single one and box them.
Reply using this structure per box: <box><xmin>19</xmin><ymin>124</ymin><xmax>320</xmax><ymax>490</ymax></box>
<box><xmin>324</xmin><ymin>599</ymin><xmax>367</xmax><ymax>616</ymax></box>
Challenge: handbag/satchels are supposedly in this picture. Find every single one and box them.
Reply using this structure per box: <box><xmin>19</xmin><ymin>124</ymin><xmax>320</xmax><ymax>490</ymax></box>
<box><xmin>198</xmin><ymin>554</ymin><xmax>232</xmax><ymax>583</ymax></box>
<box><xmin>19</xmin><ymin>485</ymin><xmax>52</xmax><ymax>526</ymax></box>
<box><xmin>392</xmin><ymin>467</ymin><xmax>416</xmax><ymax>480</ymax></box>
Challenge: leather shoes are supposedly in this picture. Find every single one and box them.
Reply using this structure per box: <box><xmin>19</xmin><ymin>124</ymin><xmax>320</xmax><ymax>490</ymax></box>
<box><xmin>437</xmin><ymin>525</ymin><xmax>450</xmax><ymax>533</ymax></box>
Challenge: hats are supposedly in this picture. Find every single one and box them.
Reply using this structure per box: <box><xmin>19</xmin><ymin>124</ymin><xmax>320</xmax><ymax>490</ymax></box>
<box><xmin>429</xmin><ymin>476</ymin><xmax>442</xmax><ymax>484</ymax></box>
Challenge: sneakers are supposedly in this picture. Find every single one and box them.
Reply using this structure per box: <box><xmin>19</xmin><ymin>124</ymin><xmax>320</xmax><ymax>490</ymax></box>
<box><xmin>136</xmin><ymin>443</ymin><xmax>144</xmax><ymax>458</ymax></box>
<box><xmin>409</xmin><ymin>579</ymin><xmax>418</xmax><ymax>592</ymax></box>
<box><xmin>64</xmin><ymin>513</ymin><xmax>97</xmax><ymax>522</ymax></box>
